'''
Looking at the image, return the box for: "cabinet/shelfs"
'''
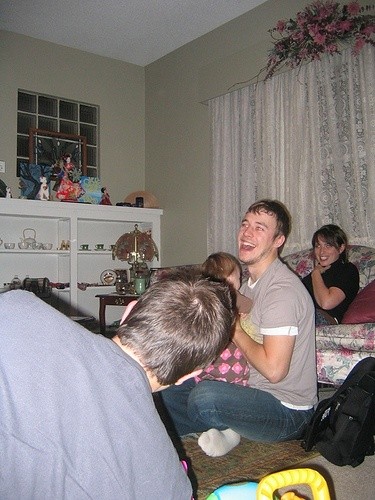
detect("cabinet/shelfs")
[0,214,161,313]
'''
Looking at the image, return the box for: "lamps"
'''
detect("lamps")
[114,221,160,287]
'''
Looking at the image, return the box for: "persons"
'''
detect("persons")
[55,153,86,203]
[100,187,111,205]
[196,251,256,387]
[0,269,233,500]
[153,199,318,456]
[297,224,361,326]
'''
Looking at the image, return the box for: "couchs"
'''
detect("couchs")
[147,244,375,386]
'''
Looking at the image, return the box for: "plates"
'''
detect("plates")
[100,270,117,285]
[108,249,114,251]
[79,249,91,250]
[94,249,106,251]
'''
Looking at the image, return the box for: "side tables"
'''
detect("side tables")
[95,293,138,335]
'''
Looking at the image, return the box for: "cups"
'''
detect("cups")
[130,285,136,294]
[117,284,125,295]
[4,283,10,291]
[136,197,143,208]
[80,245,88,250]
[135,278,145,295]
[109,245,115,249]
[95,244,104,249]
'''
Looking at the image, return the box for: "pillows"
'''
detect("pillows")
[342,279,375,325]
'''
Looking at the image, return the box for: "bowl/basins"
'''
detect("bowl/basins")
[32,242,42,249]
[4,243,15,249]
[18,242,29,249]
[42,243,52,250]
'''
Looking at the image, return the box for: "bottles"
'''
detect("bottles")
[23,275,30,288]
[66,240,71,250]
[12,275,21,285]
[61,240,66,250]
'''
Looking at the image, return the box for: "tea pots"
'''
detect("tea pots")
[19,228,37,249]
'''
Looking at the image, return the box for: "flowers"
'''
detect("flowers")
[256,0,375,80]
